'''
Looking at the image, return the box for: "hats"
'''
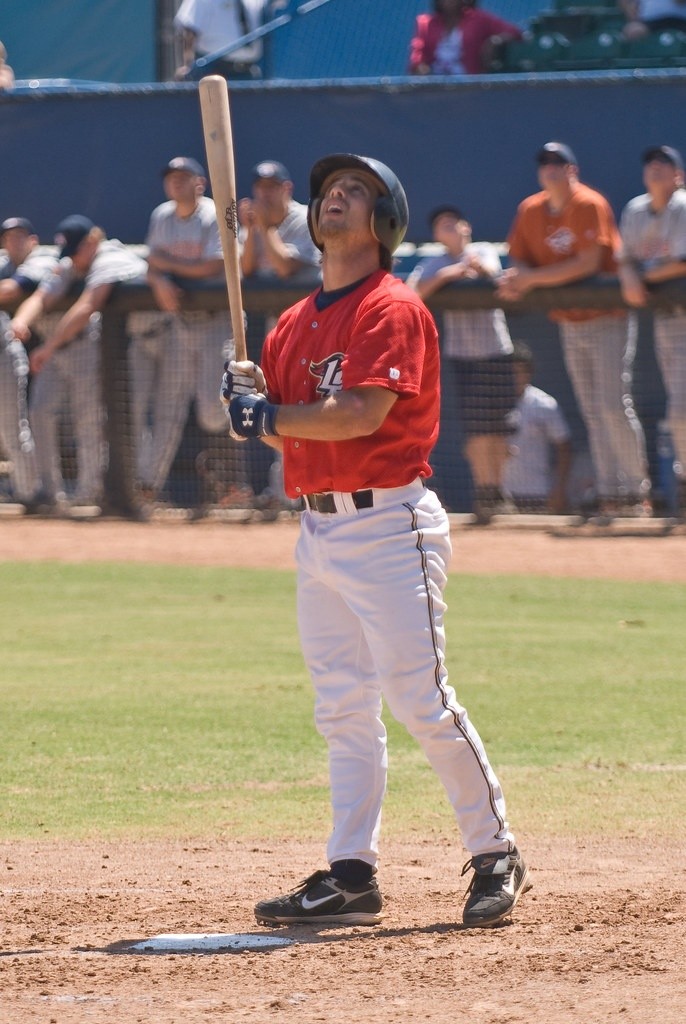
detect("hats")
[252,161,291,183]
[0,217,35,237]
[535,142,578,166]
[306,152,409,256]
[641,143,683,169]
[161,158,204,178]
[53,214,95,260]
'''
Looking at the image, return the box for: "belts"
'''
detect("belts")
[300,476,425,513]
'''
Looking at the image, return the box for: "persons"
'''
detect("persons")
[624,0,686,37]
[497,142,651,519]
[404,202,521,510]
[231,160,329,515]
[172,0,267,82]
[615,144,686,503]
[498,344,572,515]
[219,153,534,929]
[0,157,234,515]
[407,0,522,75]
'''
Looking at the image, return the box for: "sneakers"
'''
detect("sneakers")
[460,845,533,927]
[253,870,384,926]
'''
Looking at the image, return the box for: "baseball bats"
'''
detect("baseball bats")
[197,75,255,442]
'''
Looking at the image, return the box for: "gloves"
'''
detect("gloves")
[224,393,278,441]
[219,360,268,400]
